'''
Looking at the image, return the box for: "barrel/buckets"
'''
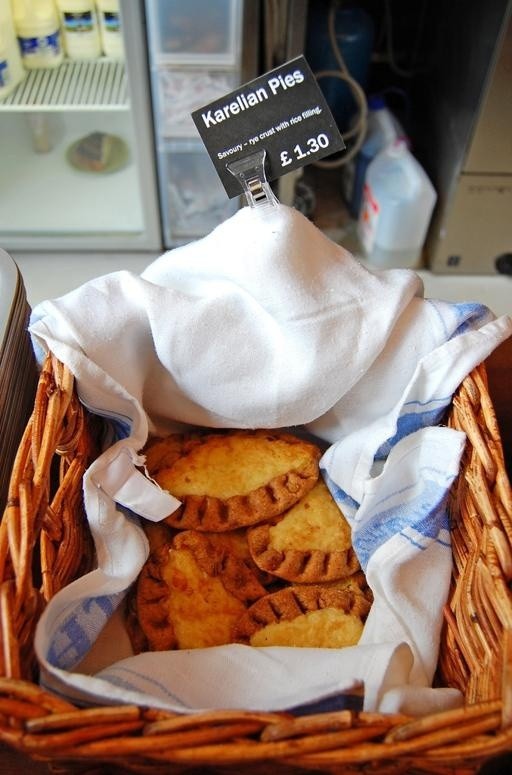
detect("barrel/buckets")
[358,141,435,276]
[311,0,376,103]
[339,103,405,218]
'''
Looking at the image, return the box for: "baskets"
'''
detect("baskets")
[1,349,511,775]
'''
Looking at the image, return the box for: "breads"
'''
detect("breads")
[124,428,374,654]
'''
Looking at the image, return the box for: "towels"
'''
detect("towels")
[25,200,512,714]
